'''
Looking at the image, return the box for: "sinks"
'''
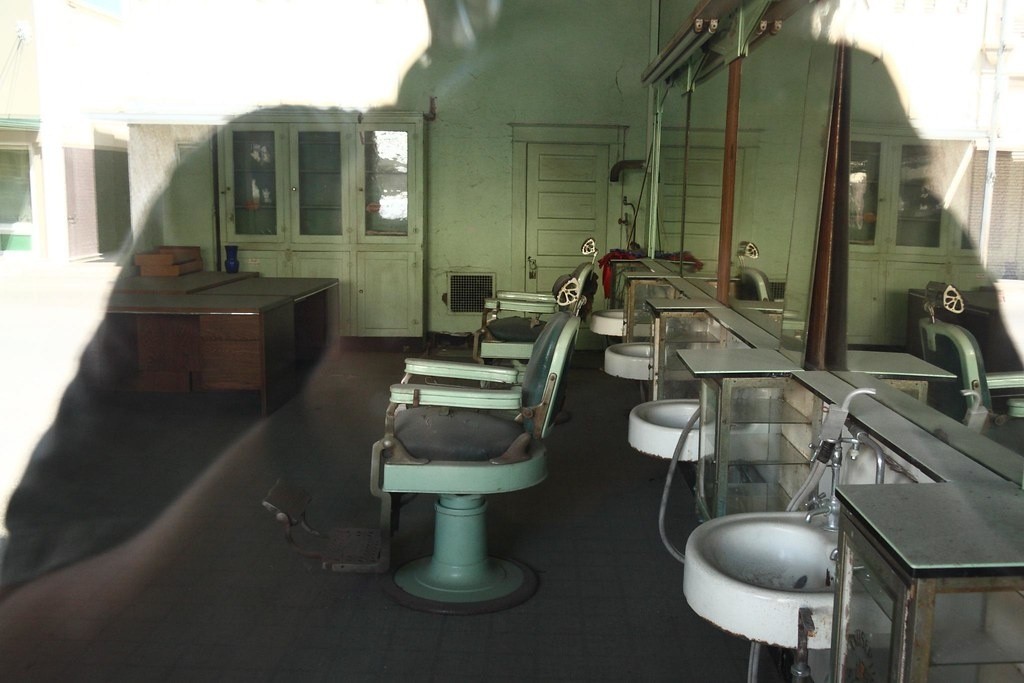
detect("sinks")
[589,309,650,337]
[604,342,693,380]
[627,398,769,462]
[681,509,950,650]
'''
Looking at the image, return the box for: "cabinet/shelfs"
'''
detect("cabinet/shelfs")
[829,481,1024,683]
[844,125,976,350]
[646,299,720,401]
[218,111,430,351]
[609,255,639,310]
[677,349,814,524]
[622,271,671,342]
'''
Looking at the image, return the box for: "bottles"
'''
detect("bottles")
[224,244,239,274]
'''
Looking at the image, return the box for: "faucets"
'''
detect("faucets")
[803,492,836,523]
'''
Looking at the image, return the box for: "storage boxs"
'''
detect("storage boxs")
[140,258,203,277]
[134,246,201,266]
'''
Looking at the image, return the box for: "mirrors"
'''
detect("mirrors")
[653,63,689,275]
[825,0,1024,488]
[681,64,730,300]
[727,0,824,339]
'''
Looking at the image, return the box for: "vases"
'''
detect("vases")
[224,245,239,272]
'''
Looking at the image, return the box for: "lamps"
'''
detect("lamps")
[640,18,719,89]
[680,19,783,97]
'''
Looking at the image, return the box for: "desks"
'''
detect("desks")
[76,292,296,419]
[76,272,261,395]
[192,277,342,396]
[902,286,1022,378]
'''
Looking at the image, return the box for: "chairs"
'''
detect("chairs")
[278,312,580,616]
[919,317,1024,434]
[472,268,598,390]
[738,267,773,302]
[392,262,593,419]
